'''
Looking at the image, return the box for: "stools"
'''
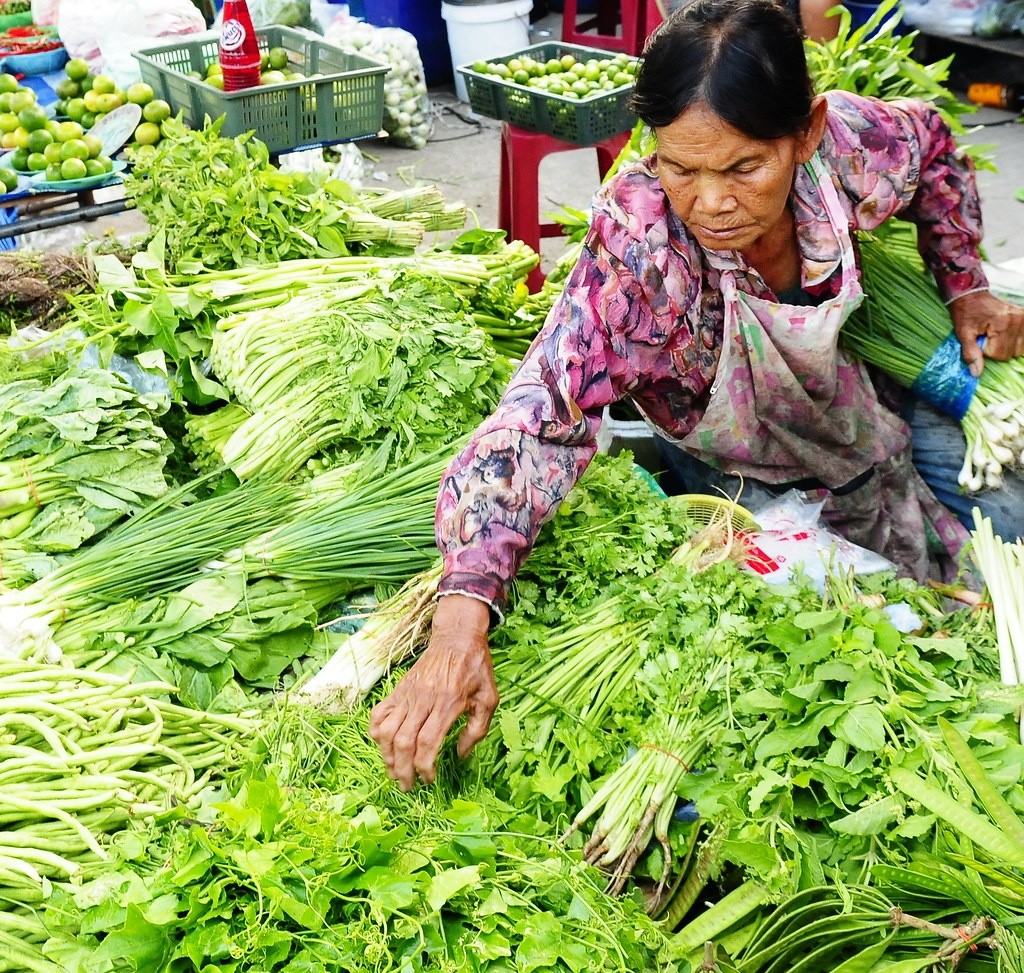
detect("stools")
[560,0,647,57]
[495,121,633,296]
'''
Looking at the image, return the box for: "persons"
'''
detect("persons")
[367,1,1024,794]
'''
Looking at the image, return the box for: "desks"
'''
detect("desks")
[0,66,152,239]
[913,30,1024,65]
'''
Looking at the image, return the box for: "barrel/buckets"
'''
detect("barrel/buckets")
[844,0,906,44]
[441,0,534,103]
[363,0,463,90]
[327,0,364,23]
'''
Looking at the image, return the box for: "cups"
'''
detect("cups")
[967,84,1012,108]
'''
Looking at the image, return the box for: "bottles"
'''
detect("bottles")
[220,0,262,120]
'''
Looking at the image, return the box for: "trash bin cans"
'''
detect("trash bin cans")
[841,0,909,47]
[441,0,535,105]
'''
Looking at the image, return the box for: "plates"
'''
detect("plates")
[86,103,143,155]
[0,152,45,175]
[116,151,137,164]
[0,175,33,201]
[31,161,126,189]
